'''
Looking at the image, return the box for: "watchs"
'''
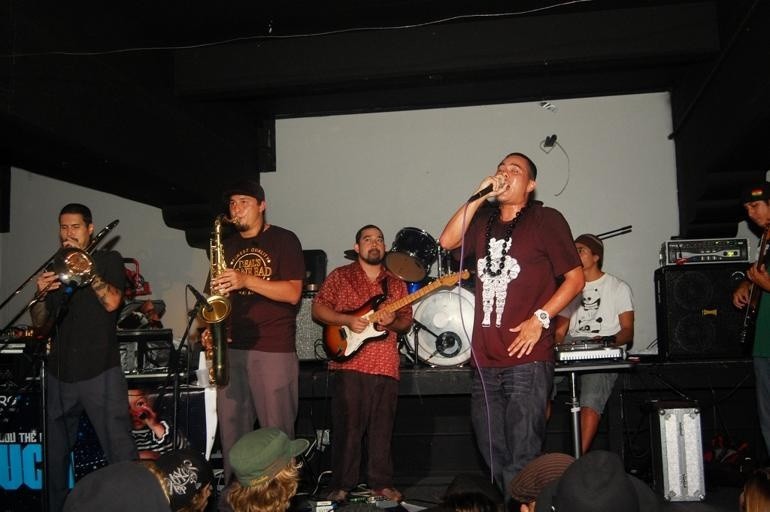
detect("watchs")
[533,308,551,330]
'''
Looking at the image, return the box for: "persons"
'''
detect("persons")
[29,202,139,512]
[217,427,311,512]
[201,181,307,487]
[310,224,414,501]
[731,181,770,453]
[439,152,586,488]
[547,233,636,454]
[509,453,576,512]
[127,385,192,462]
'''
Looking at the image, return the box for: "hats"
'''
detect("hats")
[533,450,659,512]
[228,426,310,489]
[222,180,265,201]
[574,233,605,259]
[508,452,576,503]
[743,181,770,202]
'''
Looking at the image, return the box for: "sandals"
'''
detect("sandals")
[371,486,402,502]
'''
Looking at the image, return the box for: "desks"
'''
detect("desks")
[294,372,764,478]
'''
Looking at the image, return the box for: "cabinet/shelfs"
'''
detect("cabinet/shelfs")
[116,329,182,491]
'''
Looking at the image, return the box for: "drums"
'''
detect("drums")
[384,226,437,282]
[403,285,475,367]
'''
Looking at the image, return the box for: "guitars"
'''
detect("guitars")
[738,236,770,351]
[322,269,470,363]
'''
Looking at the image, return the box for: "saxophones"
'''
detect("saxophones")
[199,213,240,388]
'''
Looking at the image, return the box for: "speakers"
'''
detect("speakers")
[653,264,756,361]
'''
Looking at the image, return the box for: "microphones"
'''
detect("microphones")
[185,284,214,315]
[63,275,82,295]
[466,184,494,206]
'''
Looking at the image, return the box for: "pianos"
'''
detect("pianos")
[552,339,634,372]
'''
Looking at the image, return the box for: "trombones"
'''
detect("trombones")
[0,218,120,352]
